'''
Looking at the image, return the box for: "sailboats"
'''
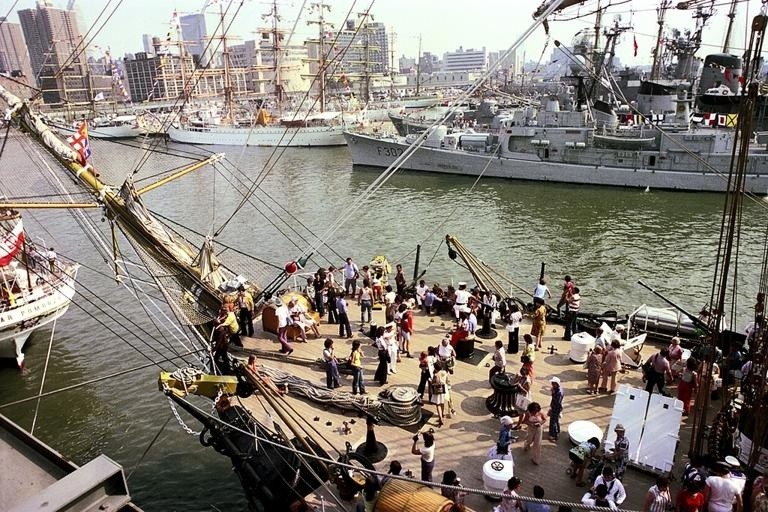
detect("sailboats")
[55,1,767,192]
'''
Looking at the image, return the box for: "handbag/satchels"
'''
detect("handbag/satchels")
[346,359,357,370]
[516,390,531,411]
[431,377,445,394]
[642,363,654,378]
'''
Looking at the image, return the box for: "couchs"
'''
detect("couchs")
[262,292,320,340]
[371,476,473,511]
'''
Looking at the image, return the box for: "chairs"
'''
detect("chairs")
[232,357,266,388]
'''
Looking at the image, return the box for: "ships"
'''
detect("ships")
[0,197,76,363]
[1,2,766,511]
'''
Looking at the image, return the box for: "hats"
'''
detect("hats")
[275,299,282,305]
[550,377,560,385]
[614,424,625,431]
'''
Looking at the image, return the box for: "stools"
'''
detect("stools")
[453,335,475,358]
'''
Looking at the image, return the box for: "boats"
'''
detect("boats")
[0,410,145,512]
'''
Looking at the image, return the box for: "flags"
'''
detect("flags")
[65,119,92,161]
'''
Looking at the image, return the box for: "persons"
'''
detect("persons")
[25,246,58,296]
[217,257,768,512]
